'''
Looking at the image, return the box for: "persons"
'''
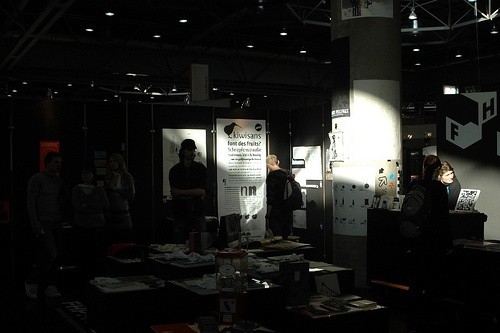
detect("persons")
[265,155,294,240]
[407,155,461,316]
[26,151,135,298]
[169,139,209,248]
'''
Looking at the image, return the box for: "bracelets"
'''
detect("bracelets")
[265,214,269,219]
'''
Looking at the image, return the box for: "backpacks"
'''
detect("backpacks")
[401,177,438,239]
[269,169,304,209]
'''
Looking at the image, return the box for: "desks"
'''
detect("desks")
[365,207,488,291]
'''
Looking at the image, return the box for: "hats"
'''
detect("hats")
[181,139,197,149]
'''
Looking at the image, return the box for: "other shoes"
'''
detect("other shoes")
[24,280,38,298]
[44,286,61,297]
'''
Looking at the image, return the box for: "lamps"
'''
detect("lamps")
[408,6,418,19]
